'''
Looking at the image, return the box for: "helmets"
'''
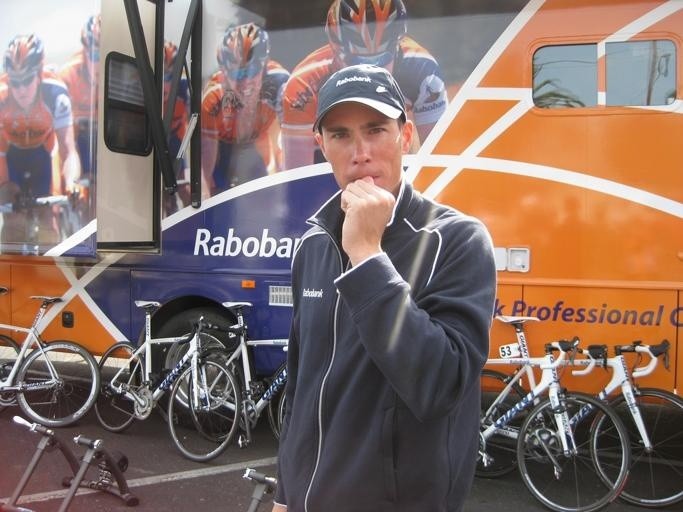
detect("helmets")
[325,0,408,55]
[164,41,177,66]
[81,15,100,46]
[216,22,270,68]
[3,32,44,76]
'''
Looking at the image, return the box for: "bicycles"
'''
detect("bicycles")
[4,193,86,259]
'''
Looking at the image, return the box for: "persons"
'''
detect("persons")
[162,42,191,216]
[201,23,289,196]
[272,65,496,512]
[282,0,448,168]
[0,15,100,243]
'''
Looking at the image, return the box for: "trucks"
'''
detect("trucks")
[1,0,683,437]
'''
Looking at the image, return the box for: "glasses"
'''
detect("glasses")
[227,69,261,80]
[10,77,35,88]
[164,72,173,81]
[89,51,100,62]
[344,52,392,66]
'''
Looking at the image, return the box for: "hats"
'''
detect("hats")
[313,64,407,133]
